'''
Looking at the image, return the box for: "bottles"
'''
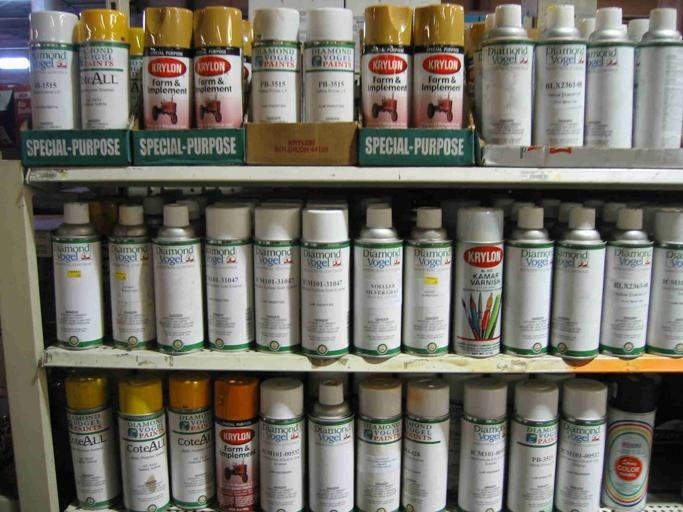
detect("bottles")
[62,369,660,512]
[52,193,682,359]
[27,2,682,151]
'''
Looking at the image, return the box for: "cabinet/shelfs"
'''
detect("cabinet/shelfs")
[0,145,683,508]
